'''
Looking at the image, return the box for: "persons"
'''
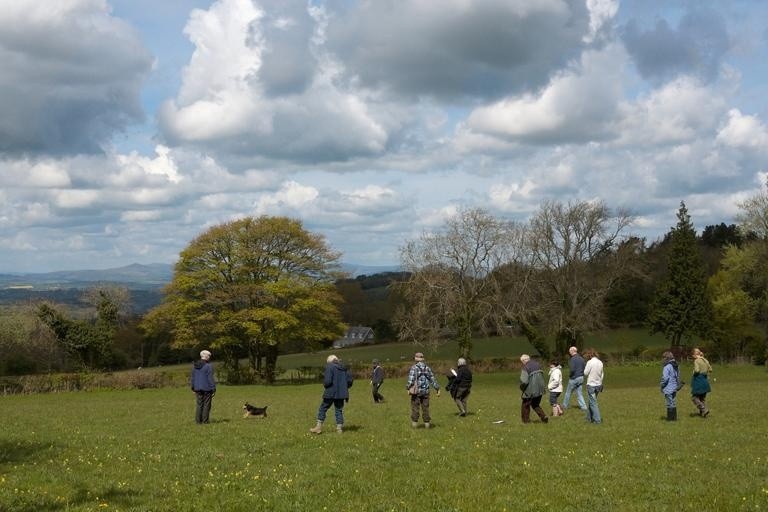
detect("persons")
[370,358,384,403]
[406,352,441,429]
[690,348,712,417]
[519,354,548,424]
[584,347,604,424]
[446,357,472,416]
[191,350,215,424]
[310,355,353,434]
[563,346,588,410]
[547,359,564,417]
[660,351,679,421]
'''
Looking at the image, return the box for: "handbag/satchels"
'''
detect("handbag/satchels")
[407,383,419,395]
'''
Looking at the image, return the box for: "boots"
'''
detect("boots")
[425,423,431,429]
[309,420,323,434]
[412,421,418,429]
[335,424,343,434]
[666,408,678,421]
[551,404,563,417]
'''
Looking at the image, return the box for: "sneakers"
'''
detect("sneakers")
[701,408,710,417]
[542,416,549,424]
[458,409,468,417]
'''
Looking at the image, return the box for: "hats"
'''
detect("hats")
[326,355,338,364]
[415,352,425,361]
[457,358,467,367]
[519,353,530,361]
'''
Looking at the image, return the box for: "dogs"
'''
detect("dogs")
[242,401,268,420]
